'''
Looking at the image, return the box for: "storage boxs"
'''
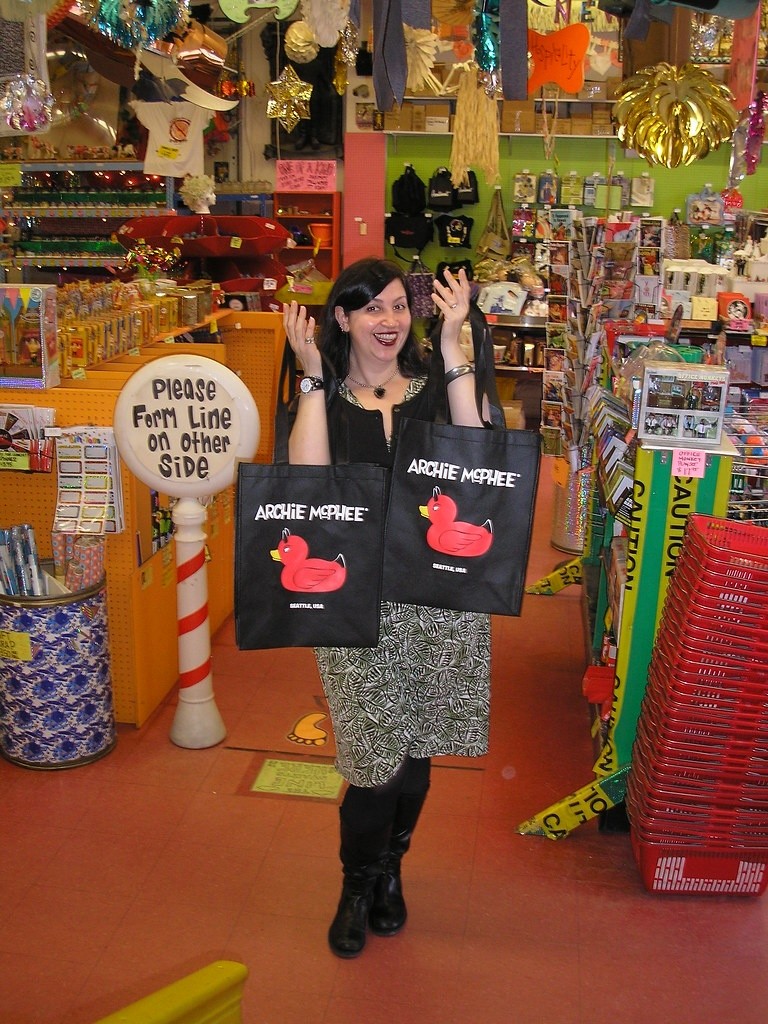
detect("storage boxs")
[577,80,608,100]
[666,290,692,319]
[412,105,425,131]
[691,296,719,322]
[425,116,449,132]
[405,61,483,97]
[569,103,592,119]
[592,104,613,136]
[501,101,535,133]
[542,82,576,99]
[552,119,571,135]
[718,292,752,319]
[729,259,768,303]
[0,283,61,389]
[637,360,730,444]
[426,101,451,117]
[571,118,592,135]
[496,377,526,429]
[535,113,553,134]
[383,103,412,132]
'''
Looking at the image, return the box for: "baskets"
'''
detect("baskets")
[625,513,768,900]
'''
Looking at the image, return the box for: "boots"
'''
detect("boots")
[368,793,426,937]
[327,807,395,959]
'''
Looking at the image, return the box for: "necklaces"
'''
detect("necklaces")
[347,365,400,399]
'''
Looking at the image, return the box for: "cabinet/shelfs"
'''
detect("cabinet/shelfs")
[517,346,768,842]
[174,193,274,218]
[0,159,174,268]
[382,96,619,155]
[273,190,342,282]
[112,215,293,312]
[0,308,287,730]
[483,312,548,372]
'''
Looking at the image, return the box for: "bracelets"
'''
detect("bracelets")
[445,363,476,386]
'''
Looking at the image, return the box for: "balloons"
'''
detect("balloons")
[0,74,55,132]
[265,65,314,133]
[612,62,741,170]
[727,90,768,190]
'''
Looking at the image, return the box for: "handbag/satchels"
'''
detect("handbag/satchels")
[234,337,387,656]
[392,165,426,216]
[434,215,474,248]
[384,212,433,263]
[402,258,436,319]
[474,190,512,261]
[427,166,478,212]
[380,301,541,616]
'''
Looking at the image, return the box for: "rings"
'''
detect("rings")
[450,303,457,309]
[305,337,314,344]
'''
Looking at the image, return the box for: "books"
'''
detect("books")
[562,213,666,528]
[540,209,577,457]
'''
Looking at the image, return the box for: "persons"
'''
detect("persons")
[282,254,492,958]
[684,415,718,438]
[645,412,678,435]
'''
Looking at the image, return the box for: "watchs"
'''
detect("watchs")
[298,375,327,395]
